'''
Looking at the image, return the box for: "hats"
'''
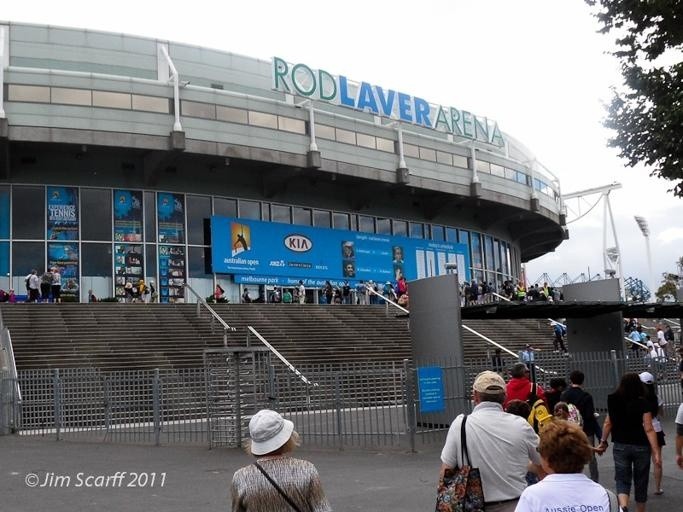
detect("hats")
[473,371,508,395]
[639,372,654,385]
[550,321,557,326]
[249,410,294,456]
[510,361,531,372]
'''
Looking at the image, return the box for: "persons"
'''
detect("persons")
[439,370,547,512]
[393,246,404,264]
[233,226,247,250]
[230,408,333,512]
[344,261,355,277]
[343,241,354,258]
[504,317,683,512]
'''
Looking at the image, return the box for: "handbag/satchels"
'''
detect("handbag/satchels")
[435,464,487,512]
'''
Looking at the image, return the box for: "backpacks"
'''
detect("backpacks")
[553,400,583,429]
[560,324,566,335]
[525,392,552,435]
